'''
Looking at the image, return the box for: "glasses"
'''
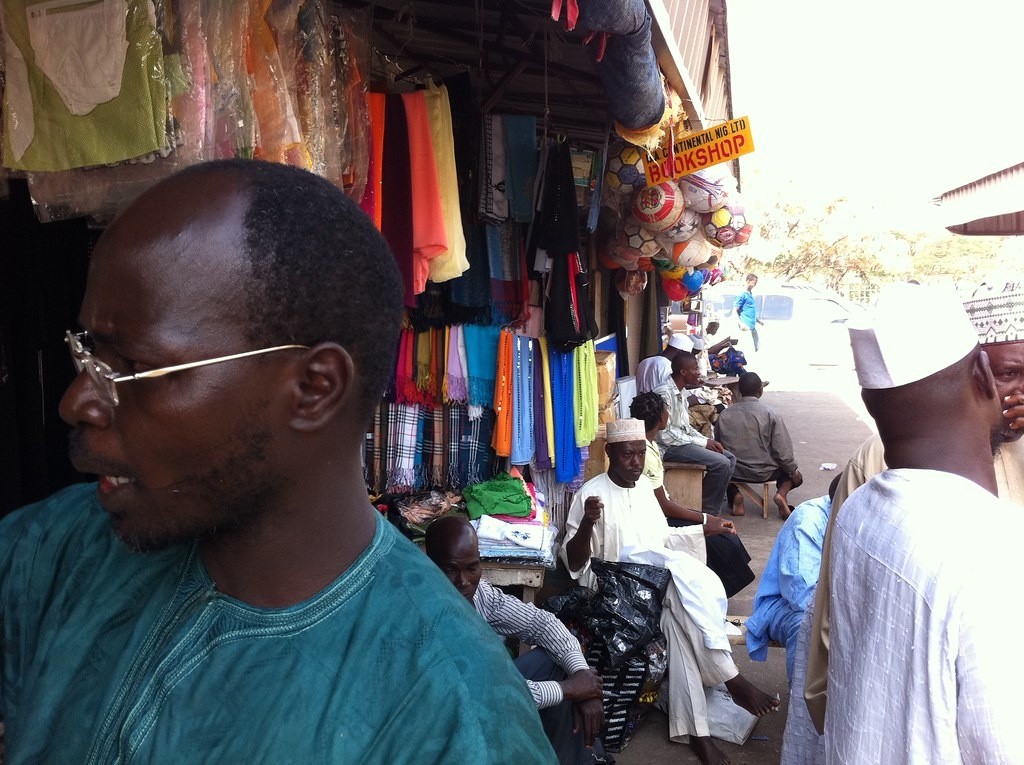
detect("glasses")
[64,328,312,407]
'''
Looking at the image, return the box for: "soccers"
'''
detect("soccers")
[603,140,757,268]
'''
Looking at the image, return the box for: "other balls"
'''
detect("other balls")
[681,269,704,290]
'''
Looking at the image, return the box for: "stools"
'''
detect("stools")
[729,481,777,519]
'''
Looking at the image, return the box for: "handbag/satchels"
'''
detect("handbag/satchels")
[589,556,671,671]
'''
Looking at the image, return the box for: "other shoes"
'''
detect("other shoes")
[762,381,769,387]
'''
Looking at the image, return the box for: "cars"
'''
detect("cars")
[727,289,860,368]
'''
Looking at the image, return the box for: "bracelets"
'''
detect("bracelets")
[701,512,709,525]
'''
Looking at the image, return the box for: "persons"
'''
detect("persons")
[422,514,605,763]
[825,285,1023,764]
[557,332,844,765]
[808,272,1023,729]
[732,274,764,353]
[0,158,561,765]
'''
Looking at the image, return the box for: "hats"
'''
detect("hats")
[688,335,703,350]
[848,282,978,389]
[606,418,647,443]
[962,283,1024,345]
[668,333,694,353]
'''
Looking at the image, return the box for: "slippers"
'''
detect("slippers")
[782,505,795,521]
